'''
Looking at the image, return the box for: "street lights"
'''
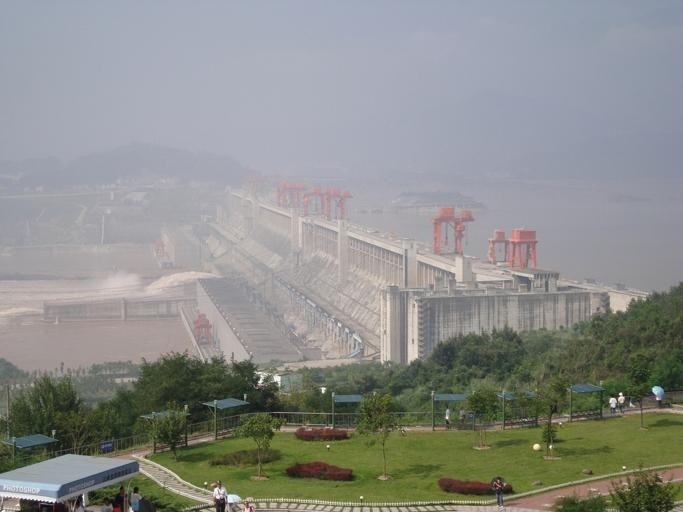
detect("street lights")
[471,389,477,432]
[598,380,605,419]
[150,410,157,454]
[242,392,249,402]
[331,390,336,429]
[11,436,17,465]
[430,390,436,431]
[51,429,57,438]
[183,404,188,447]
[213,399,217,440]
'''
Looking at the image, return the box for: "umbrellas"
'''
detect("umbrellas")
[652,385,664,397]
[225,494,241,503]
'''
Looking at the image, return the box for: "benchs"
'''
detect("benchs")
[153,427,237,453]
[433,408,602,431]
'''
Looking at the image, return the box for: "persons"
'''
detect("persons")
[116,486,131,511]
[608,394,618,416]
[225,502,235,511]
[459,407,465,425]
[445,405,452,429]
[129,487,142,512]
[618,391,626,415]
[213,480,228,511]
[493,478,505,508]
[242,501,255,512]
[656,396,662,408]
[112,502,122,512]
[73,495,85,512]
[101,497,114,512]
